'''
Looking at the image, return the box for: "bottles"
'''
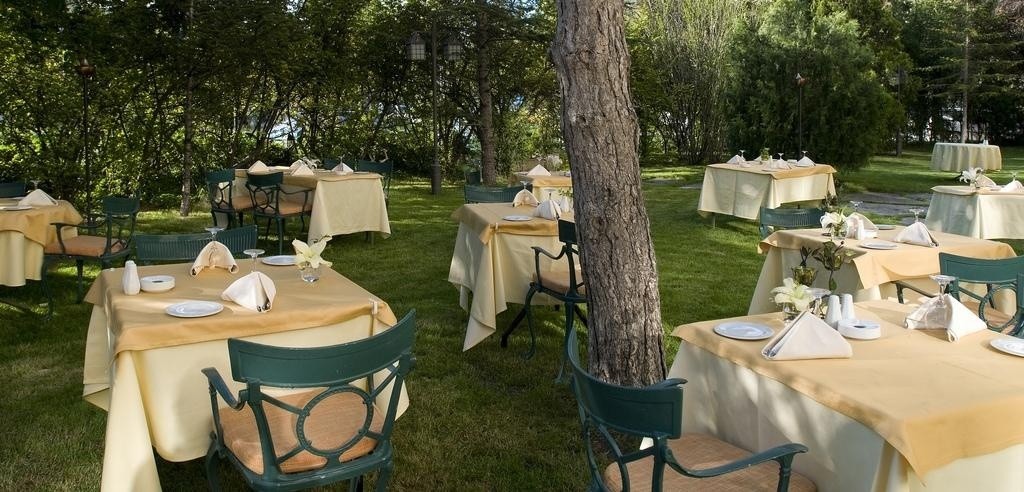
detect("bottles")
[121,259,141,296]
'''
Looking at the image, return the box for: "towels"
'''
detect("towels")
[247,160,268,173]
[289,160,308,169]
[190,240,238,275]
[331,163,354,175]
[19,188,58,207]
[290,164,314,176]
[221,272,277,312]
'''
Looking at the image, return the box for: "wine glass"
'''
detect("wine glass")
[928,274,957,337]
[29,179,41,191]
[909,208,926,225]
[1009,170,1019,183]
[337,155,346,164]
[844,199,866,238]
[982,132,990,145]
[204,226,226,273]
[739,149,746,160]
[543,188,556,201]
[770,151,808,160]
[242,249,266,276]
[536,157,543,166]
[807,286,831,320]
[301,240,322,288]
[519,180,530,190]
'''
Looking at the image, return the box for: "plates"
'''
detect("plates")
[761,167,785,173]
[854,240,902,251]
[0,205,33,211]
[165,299,223,319]
[317,168,331,174]
[353,170,371,175]
[712,321,776,341]
[502,214,534,222]
[836,317,882,341]
[871,222,897,231]
[260,254,307,266]
[138,274,176,294]
[988,335,1024,361]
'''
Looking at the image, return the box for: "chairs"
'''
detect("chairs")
[198,306,417,491]
[41,190,141,319]
[246,171,314,254]
[0,181,27,197]
[203,166,253,226]
[136,225,257,266]
[324,158,355,170]
[357,158,393,211]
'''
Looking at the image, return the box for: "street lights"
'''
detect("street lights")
[407,17,461,195]
[888,63,909,159]
[74,57,97,236]
[794,73,807,162]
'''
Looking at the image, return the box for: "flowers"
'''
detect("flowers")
[291,236,332,269]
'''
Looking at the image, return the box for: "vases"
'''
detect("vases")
[299,262,320,283]
